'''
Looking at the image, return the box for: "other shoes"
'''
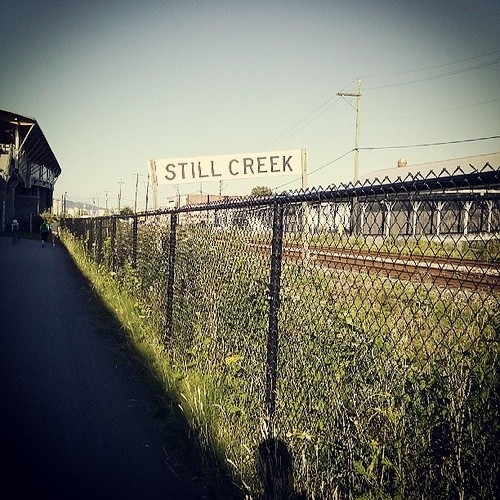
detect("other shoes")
[42,244,45,247]
[51,244,56,247]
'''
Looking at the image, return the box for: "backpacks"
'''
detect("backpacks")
[13,224,18,231]
[41,227,48,233]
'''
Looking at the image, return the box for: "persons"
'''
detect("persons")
[40,220,50,247]
[12,219,19,242]
[50,217,60,247]
